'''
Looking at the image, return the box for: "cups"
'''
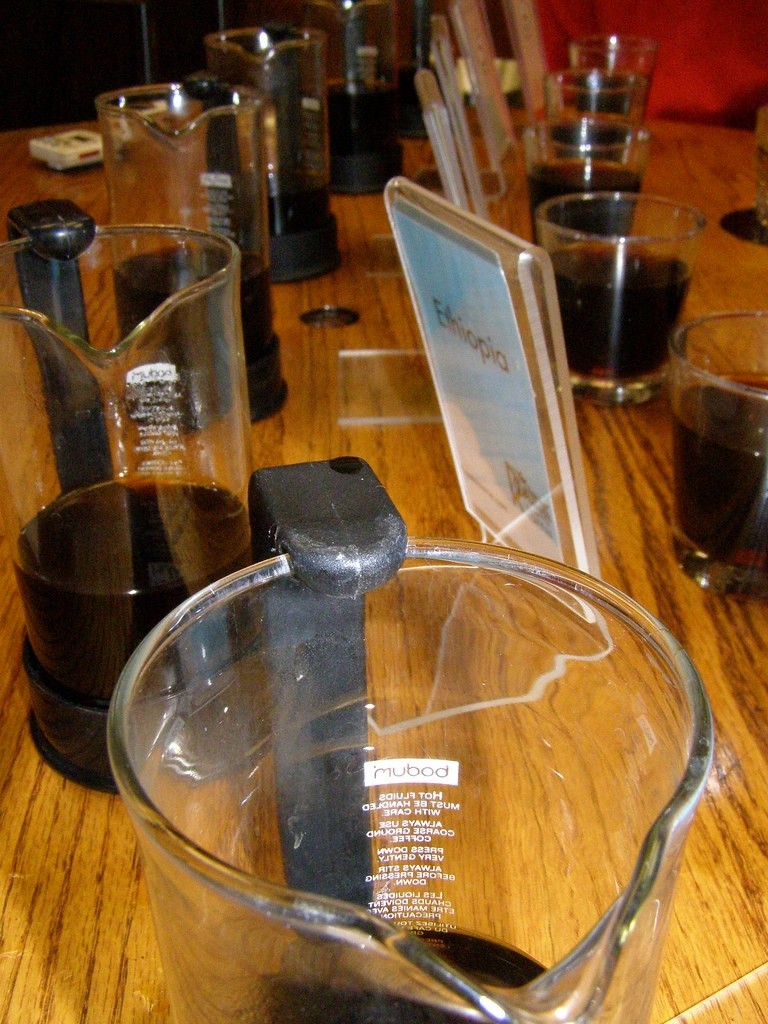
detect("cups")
[666,311,768,594]
[524,34,704,404]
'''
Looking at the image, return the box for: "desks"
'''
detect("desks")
[0,115,766,1024]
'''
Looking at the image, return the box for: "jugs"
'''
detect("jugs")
[110,458,712,1024]
[0,0,427,794]
